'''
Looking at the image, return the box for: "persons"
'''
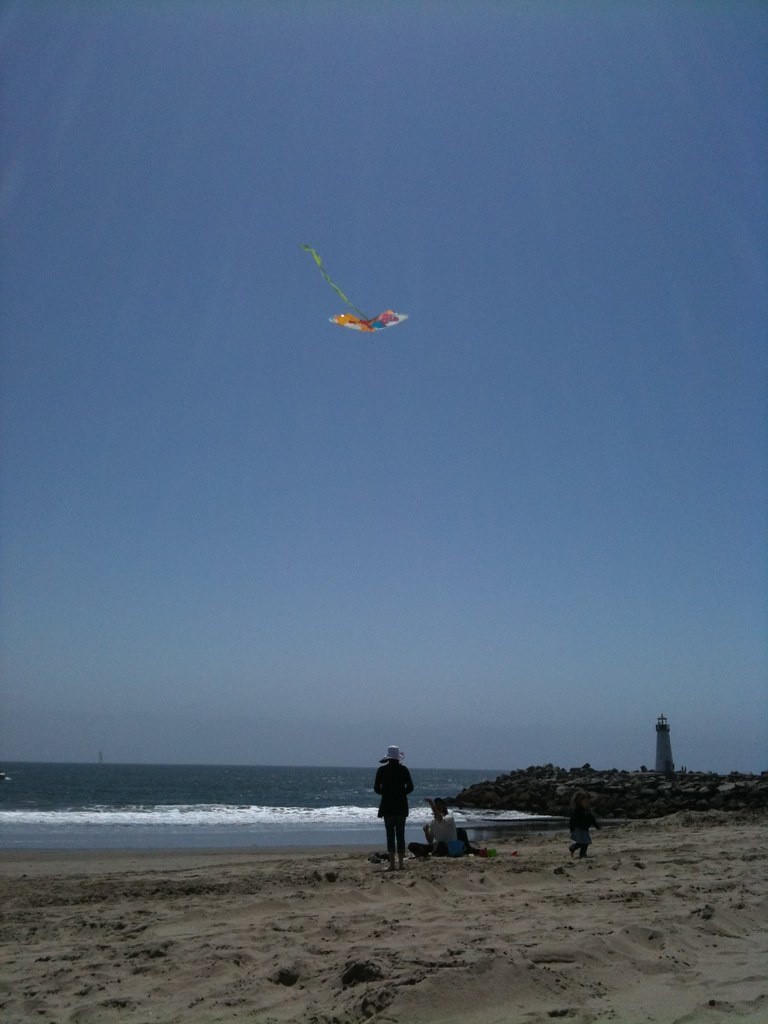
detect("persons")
[567,792,602,858]
[374,743,415,872]
[409,798,458,857]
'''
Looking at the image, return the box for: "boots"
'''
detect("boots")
[398,852,406,870]
[385,852,396,871]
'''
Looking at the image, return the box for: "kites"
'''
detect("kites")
[303,244,410,334]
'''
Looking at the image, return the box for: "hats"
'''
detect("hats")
[574,793,591,803]
[379,745,405,763]
[434,797,449,807]
